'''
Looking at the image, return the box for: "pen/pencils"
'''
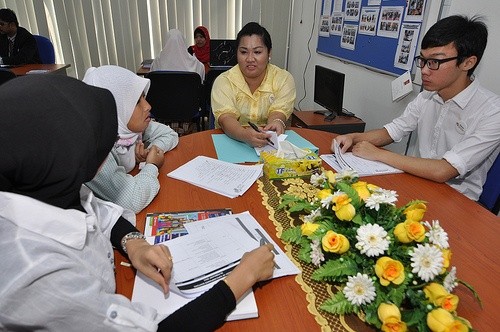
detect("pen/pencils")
[260,237,265,289]
[248,121,274,145]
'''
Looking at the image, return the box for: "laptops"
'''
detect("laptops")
[208,39,239,68]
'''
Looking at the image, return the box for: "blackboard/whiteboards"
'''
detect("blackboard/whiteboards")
[315,0,445,81]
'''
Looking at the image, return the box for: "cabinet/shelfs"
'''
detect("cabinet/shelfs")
[291,110,366,134]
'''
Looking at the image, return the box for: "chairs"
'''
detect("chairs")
[145,69,231,133]
[33,35,55,65]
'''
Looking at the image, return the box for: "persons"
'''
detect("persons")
[0,73,275,332]
[150,29,205,84]
[0,8,42,67]
[81,65,179,213]
[320,0,424,66]
[330,15,500,200]
[211,22,297,147]
[187,26,212,74]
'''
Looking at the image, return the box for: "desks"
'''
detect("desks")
[0,63,71,77]
[135,57,210,77]
[111,128,500,332]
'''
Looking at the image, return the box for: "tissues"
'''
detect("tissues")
[259,140,322,180]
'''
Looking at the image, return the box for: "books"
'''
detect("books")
[130,133,404,321]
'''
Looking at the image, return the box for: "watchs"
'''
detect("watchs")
[122,232,146,253]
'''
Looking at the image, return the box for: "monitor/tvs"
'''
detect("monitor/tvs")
[314,65,345,116]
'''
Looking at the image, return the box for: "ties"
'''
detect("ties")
[8,39,17,56]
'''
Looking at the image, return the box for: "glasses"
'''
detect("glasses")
[413,55,459,71]
[0,22,6,26]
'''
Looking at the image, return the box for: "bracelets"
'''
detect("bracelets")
[272,119,286,128]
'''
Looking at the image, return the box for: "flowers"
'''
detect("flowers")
[278,170,485,332]
